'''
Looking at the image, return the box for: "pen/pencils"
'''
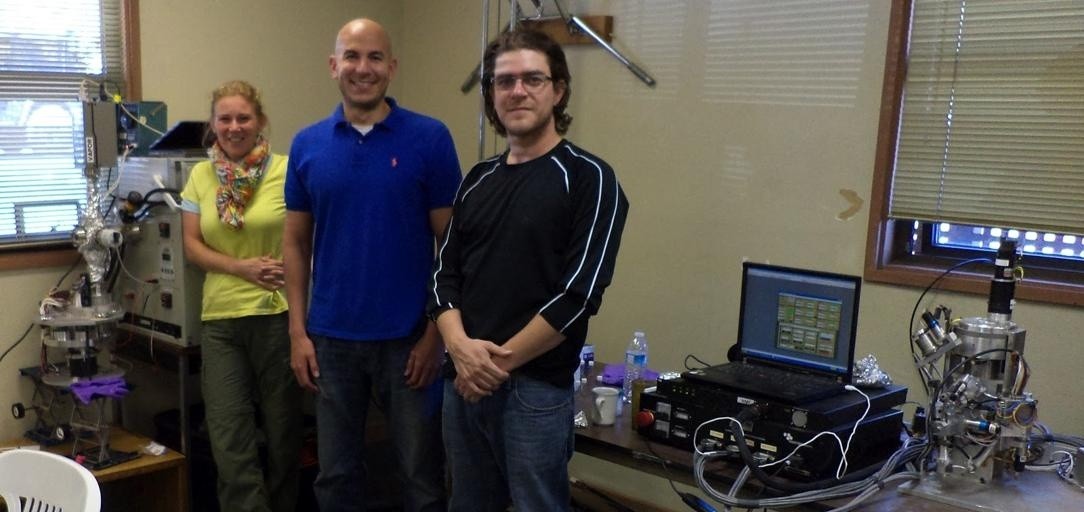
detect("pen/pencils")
[616,390,624,417]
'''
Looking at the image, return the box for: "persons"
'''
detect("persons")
[422,31,629,508]
[280,20,465,509]
[174,78,318,512]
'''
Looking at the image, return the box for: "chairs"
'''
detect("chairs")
[0,449,104,512]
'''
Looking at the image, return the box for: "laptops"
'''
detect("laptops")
[148,120,209,157]
[680,260,862,404]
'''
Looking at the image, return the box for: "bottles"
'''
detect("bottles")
[623,331,649,402]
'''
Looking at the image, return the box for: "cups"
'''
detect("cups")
[590,387,619,426]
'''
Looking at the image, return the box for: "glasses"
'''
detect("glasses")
[491,75,552,89]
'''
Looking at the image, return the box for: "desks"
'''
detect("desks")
[575,360,1084,512]
[0,422,189,512]
